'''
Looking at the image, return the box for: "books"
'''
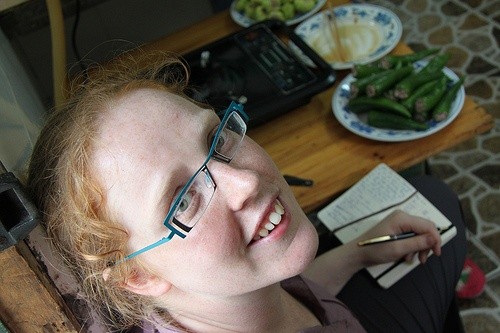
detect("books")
[316,163,458,290]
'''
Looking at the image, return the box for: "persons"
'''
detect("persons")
[28,47,469,333]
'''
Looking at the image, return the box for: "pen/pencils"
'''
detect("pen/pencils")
[356,227,441,247]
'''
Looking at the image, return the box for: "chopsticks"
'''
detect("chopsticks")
[322,2,346,64]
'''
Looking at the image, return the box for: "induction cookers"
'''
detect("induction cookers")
[127,19,334,127]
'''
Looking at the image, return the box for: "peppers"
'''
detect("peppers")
[348,49,466,132]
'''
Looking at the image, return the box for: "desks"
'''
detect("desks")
[68,0,492,217]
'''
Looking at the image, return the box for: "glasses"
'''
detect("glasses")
[107,101,248,267]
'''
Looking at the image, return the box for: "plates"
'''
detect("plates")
[295,4,403,70]
[332,57,465,141]
[230,0,325,29]
